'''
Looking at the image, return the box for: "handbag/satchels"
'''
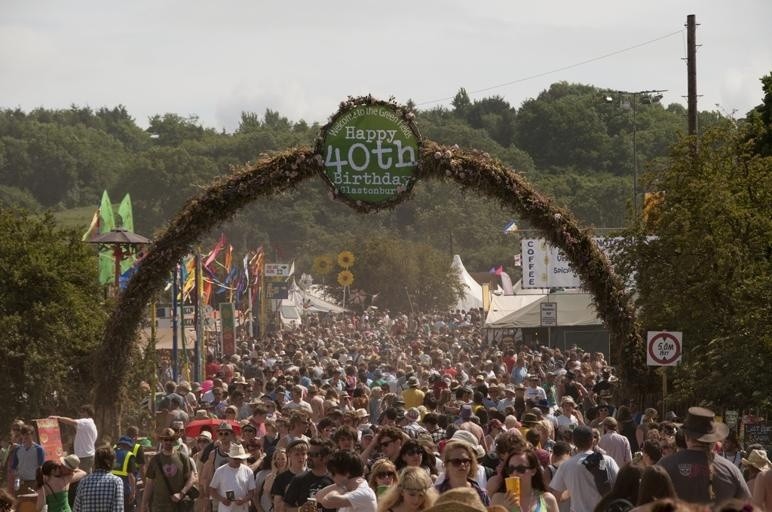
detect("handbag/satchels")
[177,495,194,510]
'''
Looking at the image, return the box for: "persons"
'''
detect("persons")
[1,357,256,511]
[212,306,619,512]
[593,403,771,512]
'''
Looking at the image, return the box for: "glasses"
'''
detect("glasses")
[377,471,394,479]
[448,458,468,466]
[508,464,530,473]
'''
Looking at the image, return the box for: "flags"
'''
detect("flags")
[481,267,513,305]
[81,190,266,304]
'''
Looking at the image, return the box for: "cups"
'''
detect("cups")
[503,477,520,507]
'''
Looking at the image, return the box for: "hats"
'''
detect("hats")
[422,487,509,512]
[118,436,133,447]
[741,449,772,471]
[60,454,80,472]
[160,346,617,459]
[673,407,729,443]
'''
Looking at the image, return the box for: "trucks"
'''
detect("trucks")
[271,298,302,332]
